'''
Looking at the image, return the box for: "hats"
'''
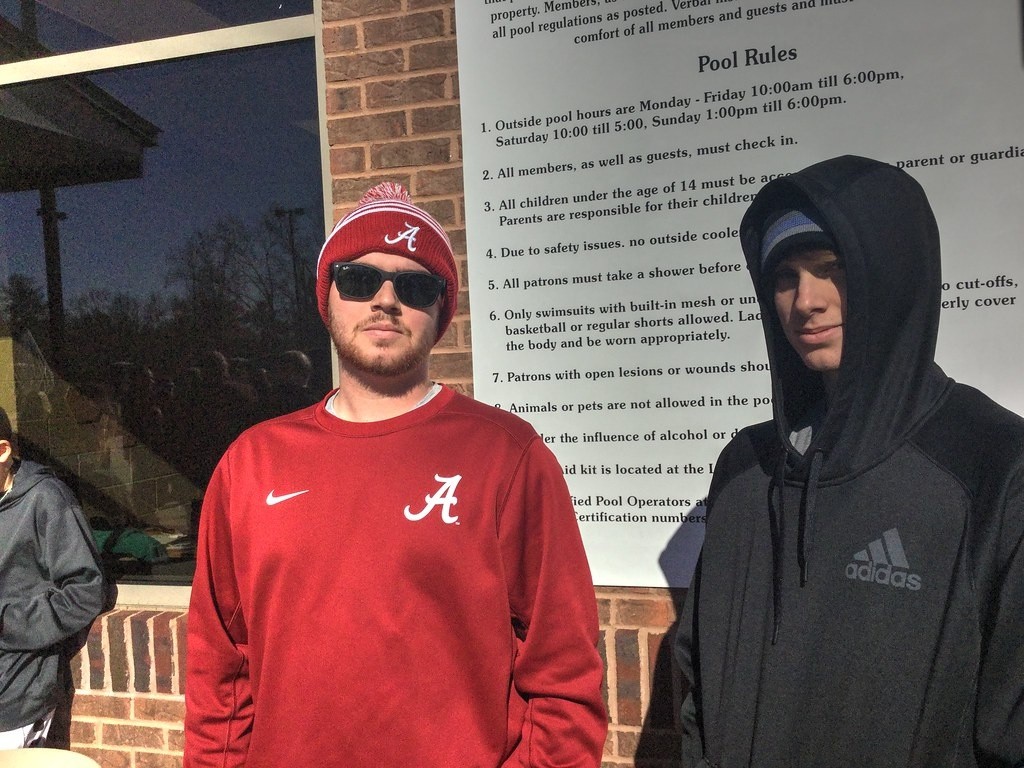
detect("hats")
[315,182,458,348]
[759,194,836,296]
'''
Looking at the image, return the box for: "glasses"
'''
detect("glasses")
[327,260,446,308]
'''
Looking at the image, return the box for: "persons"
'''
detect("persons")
[677,154,1024,767]
[113,350,321,470]
[182,182,607,768]
[0,406,105,750]
